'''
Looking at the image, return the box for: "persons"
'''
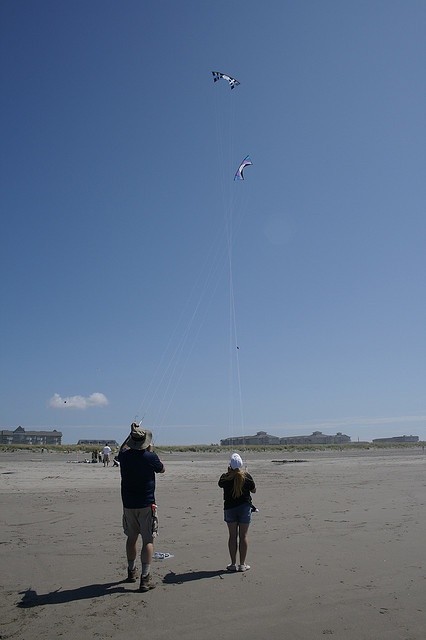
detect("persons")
[101,443,112,467]
[93,449,98,459]
[91,450,95,459]
[217,451,257,573]
[232,152,253,182]
[211,70,242,92]
[98,451,102,463]
[117,419,166,593]
[112,455,119,467]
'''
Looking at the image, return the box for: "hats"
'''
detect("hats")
[231,453,243,469]
[126,428,152,451]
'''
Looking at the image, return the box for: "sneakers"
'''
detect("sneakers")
[227,564,236,571]
[128,566,140,582]
[139,572,156,592]
[238,564,250,572]
